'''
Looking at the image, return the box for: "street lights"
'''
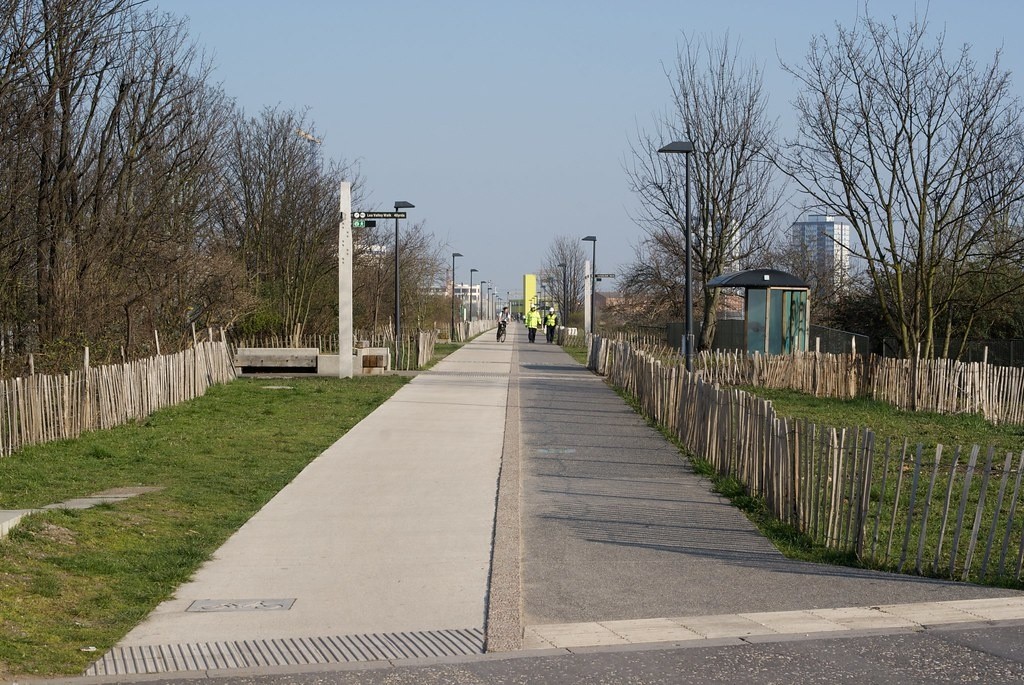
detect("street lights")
[480,281,487,320]
[537,291,542,313]
[495,295,498,319]
[451,253,464,341]
[532,298,535,305]
[488,288,493,320]
[470,268,478,321]
[534,295,538,305]
[500,299,503,311]
[547,276,554,307]
[658,140,694,375]
[394,200,416,369]
[558,263,567,327]
[540,286,546,328]
[492,293,496,320]
[582,236,597,333]
[498,298,500,310]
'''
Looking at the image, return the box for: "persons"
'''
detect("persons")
[496,307,511,342]
[544,307,558,344]
[525,302,542,343]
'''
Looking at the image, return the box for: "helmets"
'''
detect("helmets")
[530,304,535,308]
[549,307,554,312]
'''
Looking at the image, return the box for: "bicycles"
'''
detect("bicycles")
[497,323,508,343]
